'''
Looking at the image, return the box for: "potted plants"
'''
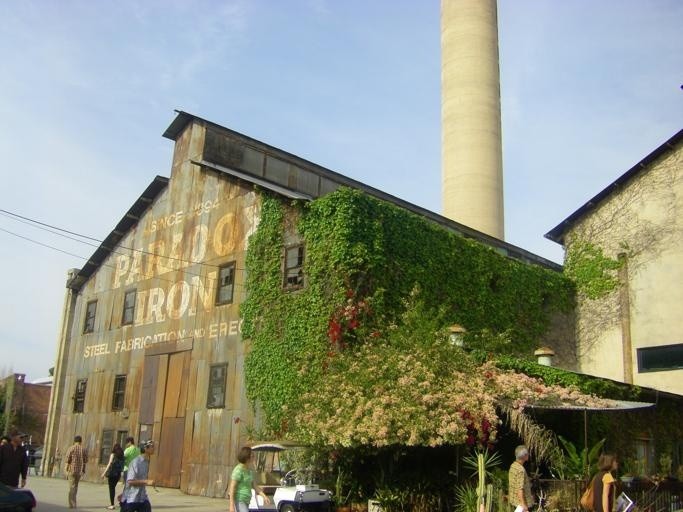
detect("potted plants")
[330,465,402,512]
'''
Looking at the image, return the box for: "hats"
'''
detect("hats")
[9,431,28,439]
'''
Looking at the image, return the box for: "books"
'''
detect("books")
[615,492,632,512]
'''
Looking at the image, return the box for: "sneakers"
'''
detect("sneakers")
[106,505,115,509]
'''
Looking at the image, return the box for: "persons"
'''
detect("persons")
[508,444,534,511]
[591,453,619,511]
[0,431,29,489]
[0,436,10,446]
[100,443,123,510]
[64,436,87,508]
[123,437,140,490]
[227,447,270,511]
[119,439,154,511]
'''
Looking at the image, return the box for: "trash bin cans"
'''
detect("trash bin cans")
[368,499,387,512]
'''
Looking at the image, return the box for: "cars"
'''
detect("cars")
[28,445,44,468]
